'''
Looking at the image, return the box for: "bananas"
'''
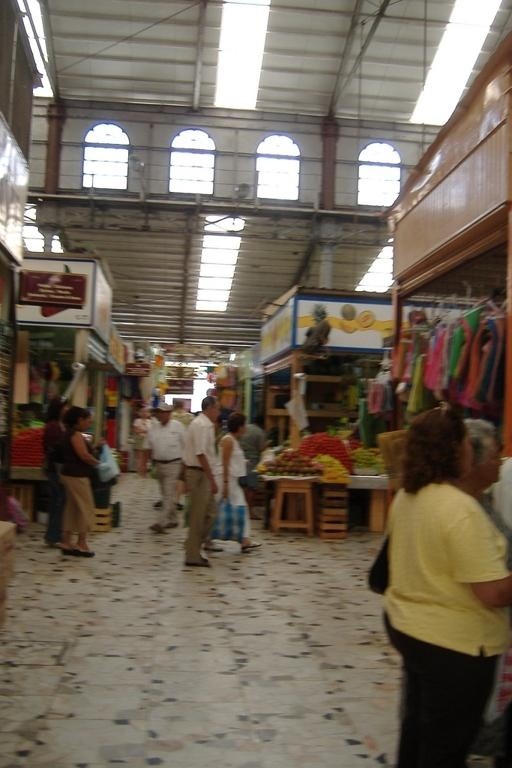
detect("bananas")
[256,464,268,473]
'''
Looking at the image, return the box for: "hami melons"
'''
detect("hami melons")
[342,304,356,320]
[358,310,374,328]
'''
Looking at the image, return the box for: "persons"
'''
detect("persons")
[41,392,72,547]
[134,397,227,512]
[204,412,262,553]
[141,399,187,533]
[55,403,107,557]
[380,404,511,768]
[456,416,511,568]
[239,415,268,522]
[180,395,219,567]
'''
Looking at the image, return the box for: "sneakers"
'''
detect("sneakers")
[149,499,183,533]
[185,542,262,567]
[43,537,95,557]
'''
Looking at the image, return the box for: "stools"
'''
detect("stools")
[267,478,315,538]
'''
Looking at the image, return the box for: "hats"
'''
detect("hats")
[153,402,175,412]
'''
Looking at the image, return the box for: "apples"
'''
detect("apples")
[350,447,377,468]
[264,449,321,476]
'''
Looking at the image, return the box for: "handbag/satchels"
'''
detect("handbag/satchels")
[96,444,120,482]
[368,535,389,593]
